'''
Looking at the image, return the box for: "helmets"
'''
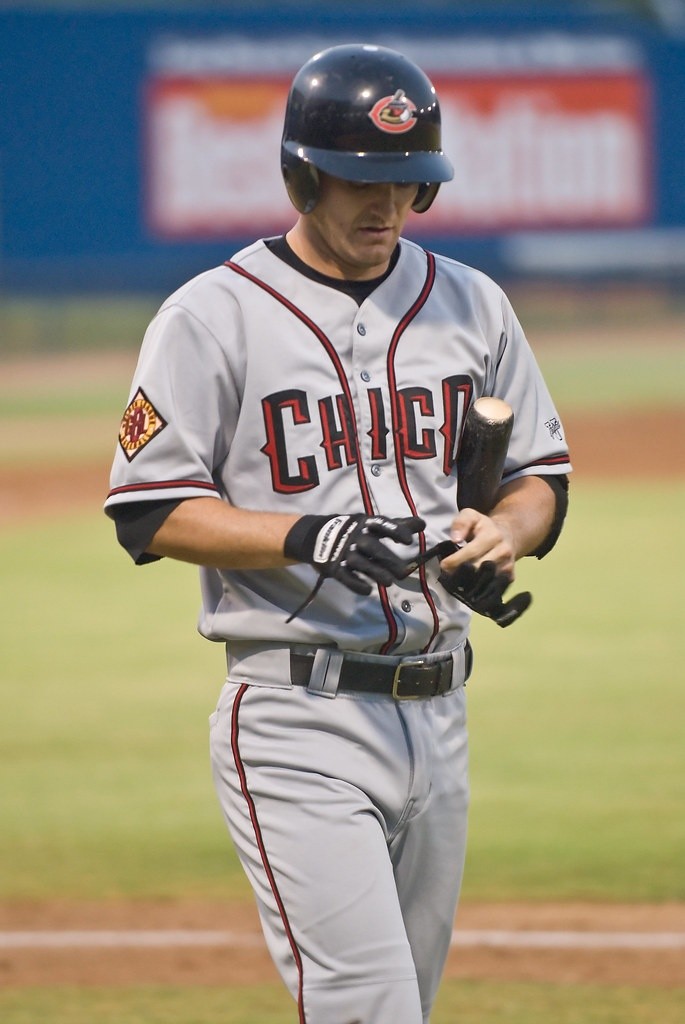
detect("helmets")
[280,43,453,214]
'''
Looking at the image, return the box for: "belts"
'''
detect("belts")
[290,637,473,701]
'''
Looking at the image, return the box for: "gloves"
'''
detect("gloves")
[397,540,531,629]
[284,514,426,596]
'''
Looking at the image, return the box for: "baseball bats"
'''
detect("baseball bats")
[458,396,514,517]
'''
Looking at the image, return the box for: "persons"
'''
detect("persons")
[103,46,577,1024]
